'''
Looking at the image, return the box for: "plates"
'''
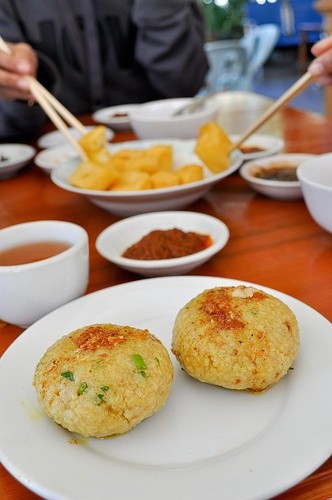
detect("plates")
[240,154,319,199]
[37,125,114,147]
[92,104,141,130]
[183,135,285,170]
[0,143,36,179]
[95,210,230,278]
[0,275,331,500]
[33,142,112,174]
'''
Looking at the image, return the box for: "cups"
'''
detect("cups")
[1,220,89,329]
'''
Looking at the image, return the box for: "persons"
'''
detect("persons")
[0,0,212,143]
[310,0,331,90]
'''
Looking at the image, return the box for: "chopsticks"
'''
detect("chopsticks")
[0,36,90,165]
[226,72,317,155]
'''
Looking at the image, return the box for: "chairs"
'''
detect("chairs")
[201,24,280,93]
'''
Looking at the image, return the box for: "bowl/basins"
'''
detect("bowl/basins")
[296,152,332,234]
[49,138,245,217]
[127,97,220,141]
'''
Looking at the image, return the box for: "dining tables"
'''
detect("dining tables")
[0,88,331,500]
